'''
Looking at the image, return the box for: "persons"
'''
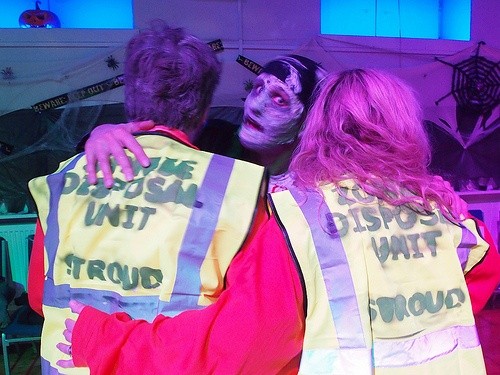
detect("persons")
[82,53,330,187]
[51,65,498,375]
[24,11,270,375]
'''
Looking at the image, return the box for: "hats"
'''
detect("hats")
[260,55,330,111]
